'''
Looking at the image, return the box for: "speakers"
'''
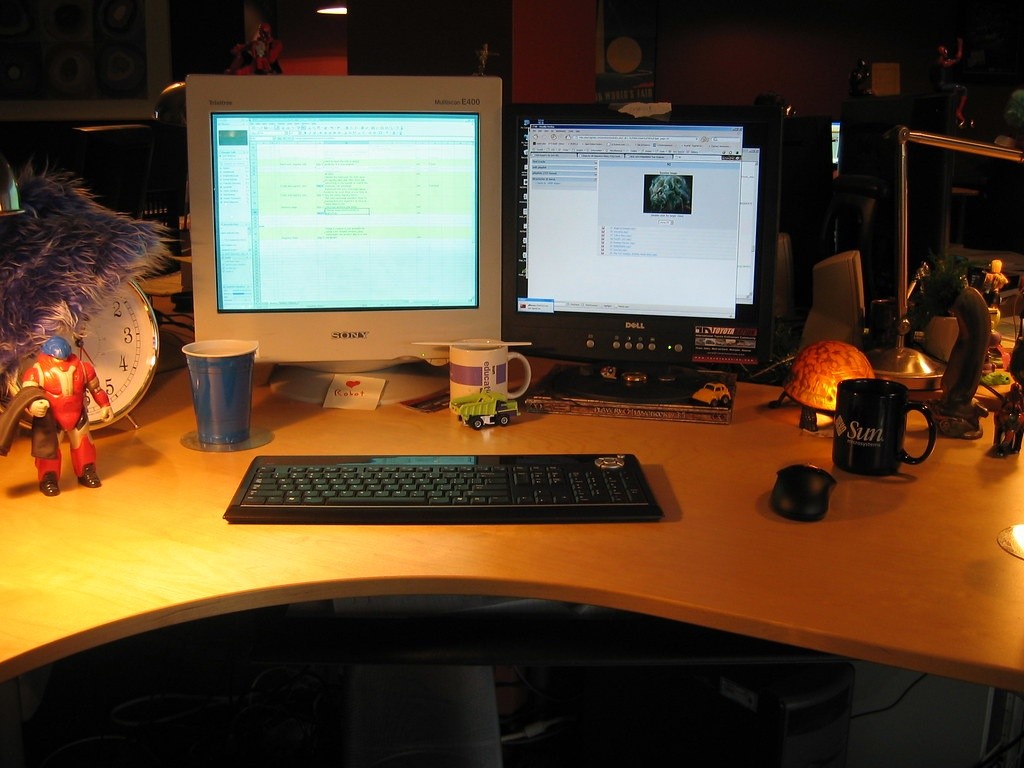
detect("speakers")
[797,251,866,356]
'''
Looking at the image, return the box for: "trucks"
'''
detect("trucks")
[449,389,521,430]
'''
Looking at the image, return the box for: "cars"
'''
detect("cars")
[690,381,732,407]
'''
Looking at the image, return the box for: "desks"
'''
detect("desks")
[0,363,1024,768]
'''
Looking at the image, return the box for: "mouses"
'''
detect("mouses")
[770,464,837,522]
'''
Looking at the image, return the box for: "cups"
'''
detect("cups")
[832,378,937,477]
[182,338,259,445]
[449,339,532,415]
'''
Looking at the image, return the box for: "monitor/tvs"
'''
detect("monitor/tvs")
[185,73,503,408]
[500,102,787,406]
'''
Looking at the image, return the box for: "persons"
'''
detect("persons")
[225,24,284,74]
[20,336,114,495]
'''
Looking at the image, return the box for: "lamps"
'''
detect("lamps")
[785,340,876,431]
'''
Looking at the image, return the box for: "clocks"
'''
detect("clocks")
[0,273,161,432]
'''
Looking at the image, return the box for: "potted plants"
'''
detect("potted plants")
[965,259,1002,331]
[922,248,975,360]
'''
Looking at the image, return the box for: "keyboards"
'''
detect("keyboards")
[223,454,664,525]
